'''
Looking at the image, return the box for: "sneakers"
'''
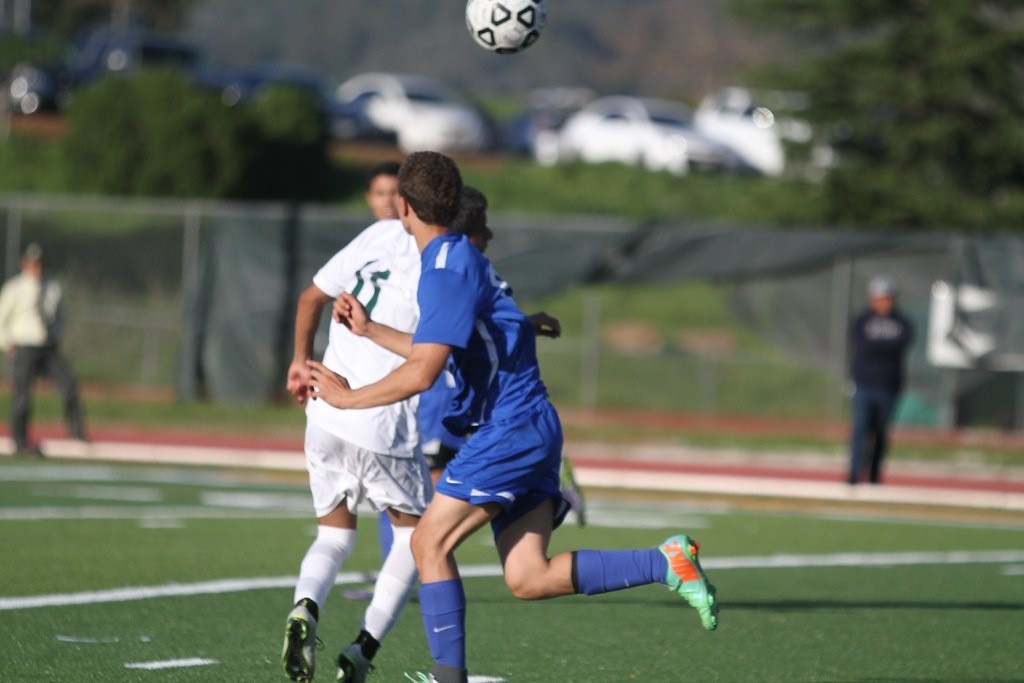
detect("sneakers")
[280,598,324,683]
[334,629,381,683]
[657,534,720,631]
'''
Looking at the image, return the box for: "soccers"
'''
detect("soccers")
[465,0,548,55]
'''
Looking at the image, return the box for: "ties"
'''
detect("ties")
[33,286,49,330]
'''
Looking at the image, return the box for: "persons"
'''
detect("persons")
[0,241,82,456]
[307,151,718,683]
[281,162,586,683]
[847,277,913,483]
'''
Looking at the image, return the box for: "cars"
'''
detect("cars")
[13,33,339,144]
[336,72,491,155]
[700,88,812,180]
[521,91,746,179]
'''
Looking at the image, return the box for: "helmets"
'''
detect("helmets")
[865,264,900,297]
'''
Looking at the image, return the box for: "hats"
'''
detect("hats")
[18,241,46,260]
